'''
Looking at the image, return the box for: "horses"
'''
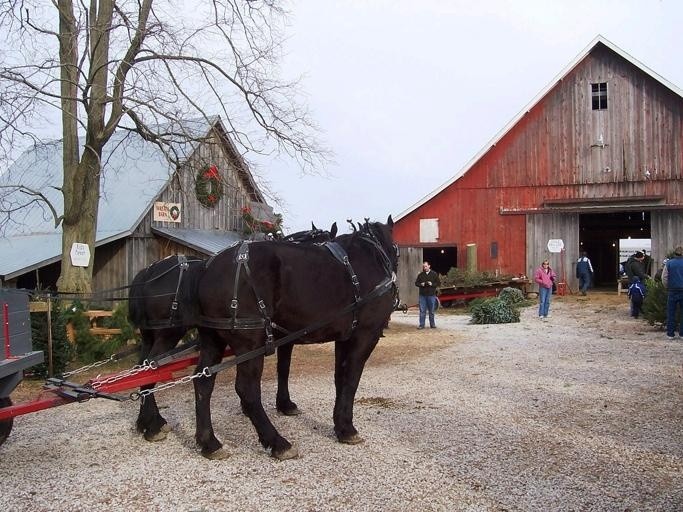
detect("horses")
[181,215,400,463]
[126,222,341,442]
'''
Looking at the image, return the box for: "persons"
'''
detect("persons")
[625,250,649,281]
[654,258,668,282]
[414,261,440,330]
[576,252,594,295]
[534,259,555,318]
[661,247,682,339]
[626,275,646,317]
